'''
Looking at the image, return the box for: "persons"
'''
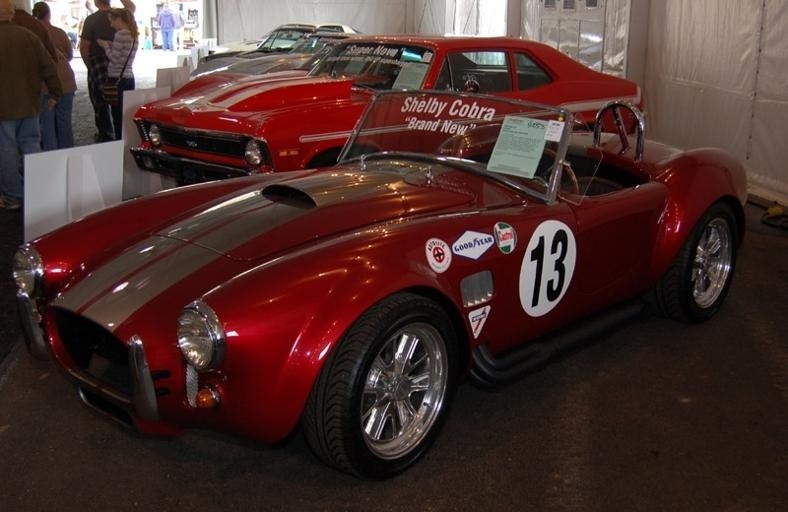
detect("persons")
[32,0,73,62]
[1,1,63,210]
[40,48,77,149]
[80,0,135,141]
[11,4,59,92]
[96,8,139,141]
[158,3,177,51]
[173,3,186,51]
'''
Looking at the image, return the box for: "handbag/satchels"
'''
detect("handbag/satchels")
[103,35,136,102]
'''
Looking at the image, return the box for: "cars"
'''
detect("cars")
[129,35,645,177]
[187,26,340,81]
[226,34,354,75]
[13,89,748,482]
[205,22,361,56]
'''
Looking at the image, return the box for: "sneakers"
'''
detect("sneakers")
[0,195,21,209]
